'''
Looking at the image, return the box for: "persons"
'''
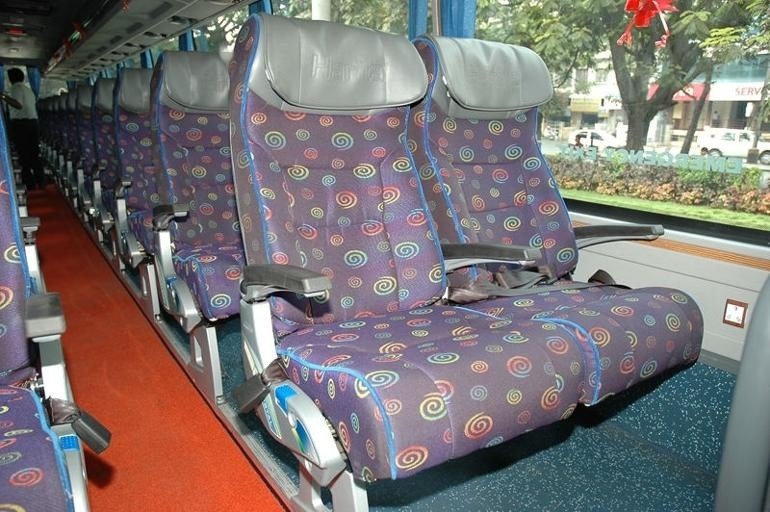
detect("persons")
[0,69,45,190]
[573,134,584,148]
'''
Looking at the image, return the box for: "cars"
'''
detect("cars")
[696,128,770,167]
[568,129,622,150]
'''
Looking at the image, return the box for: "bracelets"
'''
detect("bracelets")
[2,94,6,100]
